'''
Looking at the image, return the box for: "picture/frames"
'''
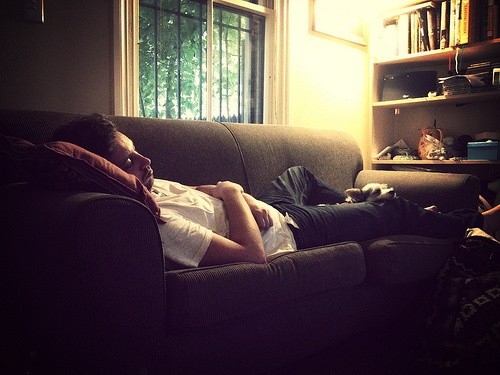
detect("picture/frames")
[309,0,370,52]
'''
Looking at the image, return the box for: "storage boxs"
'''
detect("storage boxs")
[467,141,499,160]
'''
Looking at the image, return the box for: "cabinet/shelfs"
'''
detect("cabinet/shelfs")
[369,0,500,237]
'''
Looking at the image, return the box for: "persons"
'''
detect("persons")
[54,112,500,268]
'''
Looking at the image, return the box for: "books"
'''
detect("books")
[384,0,494,56]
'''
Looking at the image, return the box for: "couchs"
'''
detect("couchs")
[0,108,485,375]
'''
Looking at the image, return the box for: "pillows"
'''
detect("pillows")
[0,134,168,224]
